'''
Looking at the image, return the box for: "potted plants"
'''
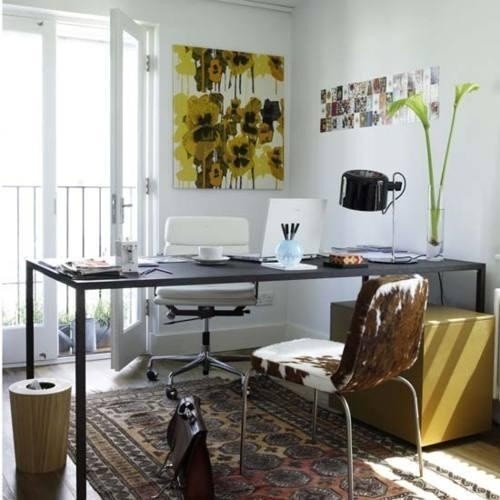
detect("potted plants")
[386,82,480,258]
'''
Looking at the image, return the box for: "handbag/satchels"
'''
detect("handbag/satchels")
[167,393,215,499]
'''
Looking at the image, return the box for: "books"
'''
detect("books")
[331,245,409,259]
[38,256,159,280]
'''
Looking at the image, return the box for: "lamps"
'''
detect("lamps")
[339,167,420,263]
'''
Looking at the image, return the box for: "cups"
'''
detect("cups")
[197,245,224,261]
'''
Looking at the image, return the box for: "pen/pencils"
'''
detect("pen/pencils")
[281,223,300,240]
[141,268,172,275]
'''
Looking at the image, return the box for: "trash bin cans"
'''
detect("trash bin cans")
[9,378,72,473]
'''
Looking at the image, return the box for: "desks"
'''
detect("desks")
[26,251,487,500]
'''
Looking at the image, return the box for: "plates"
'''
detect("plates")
[190,254,232,266]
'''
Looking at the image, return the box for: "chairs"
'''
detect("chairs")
[148,217,251,400]
[245,274,432,500]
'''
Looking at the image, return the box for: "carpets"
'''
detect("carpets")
[66,371,500,500]
